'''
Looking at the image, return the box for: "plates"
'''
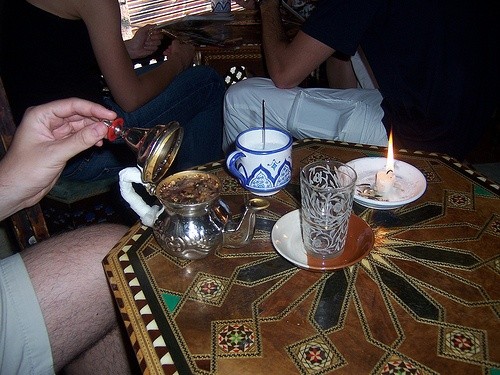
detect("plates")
[338,156,427,209]
[271,208,375,270]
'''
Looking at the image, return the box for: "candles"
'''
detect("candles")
[377,129,394,195]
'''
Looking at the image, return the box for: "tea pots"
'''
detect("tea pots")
[97,118,270,260]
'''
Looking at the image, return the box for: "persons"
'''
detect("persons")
[222,0,492,159]
[0,101,153,375]
[0,0,222,181]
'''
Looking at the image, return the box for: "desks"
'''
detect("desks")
[158,11,329,88]
[102,138,500,375]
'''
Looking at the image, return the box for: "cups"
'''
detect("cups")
[226,127,293,197]
[212,1,232,15]
[300,159,358,260]
